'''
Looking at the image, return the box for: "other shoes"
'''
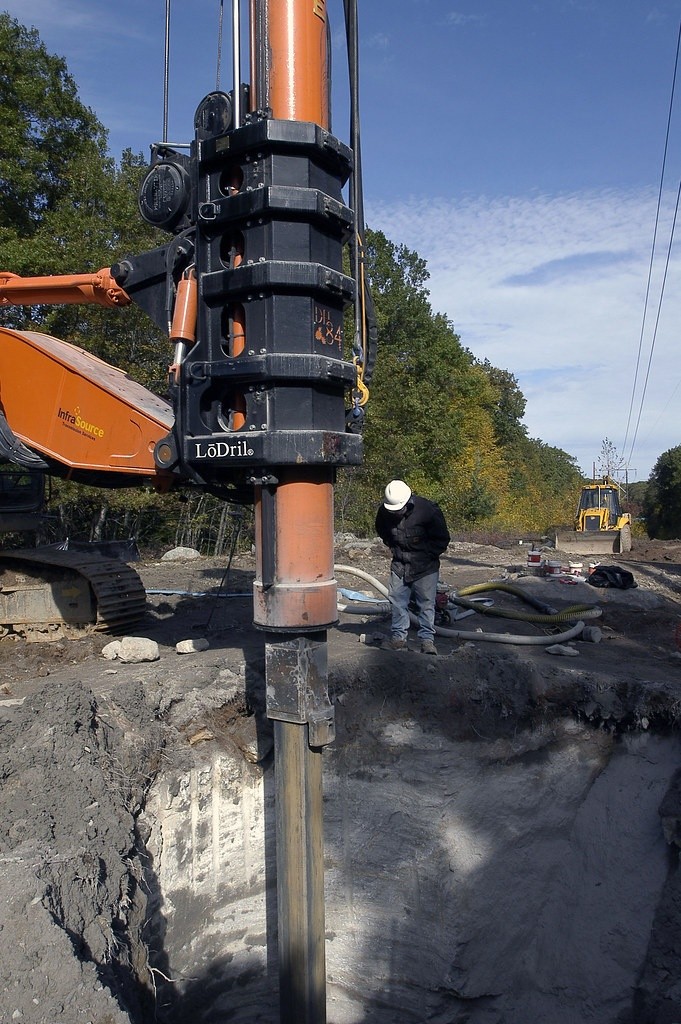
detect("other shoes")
[380,641,408,652]
[420,640,437,655]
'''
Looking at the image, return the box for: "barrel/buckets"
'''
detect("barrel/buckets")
[544,560,549,570]
[569,561,583,574]
[588,562,602,574]
[549,561,562,577]
[527,551,542,566]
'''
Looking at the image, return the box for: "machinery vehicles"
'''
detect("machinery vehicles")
[554,483,633,555]
[0,0,366,1023]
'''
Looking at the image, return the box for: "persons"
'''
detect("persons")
[374,478,450,654]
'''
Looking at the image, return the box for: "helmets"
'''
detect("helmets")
[384,480,411,510]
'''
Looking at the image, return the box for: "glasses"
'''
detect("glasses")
[388,506,405,513]
